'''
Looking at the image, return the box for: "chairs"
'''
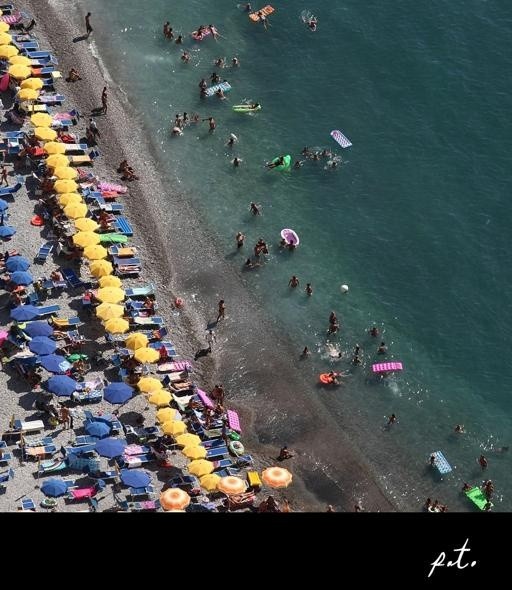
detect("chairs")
[0,0,262,513]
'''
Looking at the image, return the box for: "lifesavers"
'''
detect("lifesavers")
[230,440,245,454]
[272,155,292,171]
[301,9,315,22]
[320,373,332,384]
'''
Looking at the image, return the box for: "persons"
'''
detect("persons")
[1,1,500,513]
